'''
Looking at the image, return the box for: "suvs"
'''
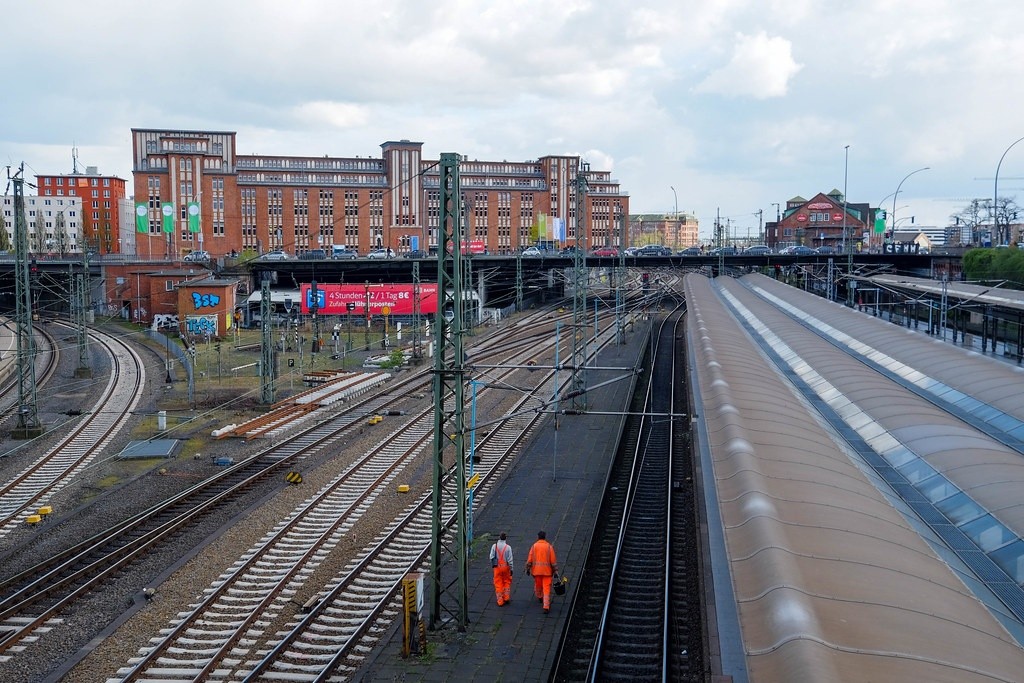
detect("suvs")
[632,245,666,257]
[298,249,327,260]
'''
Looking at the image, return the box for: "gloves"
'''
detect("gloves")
[526,570,530,576]
[554,574,558,579]
[510,571,513,576]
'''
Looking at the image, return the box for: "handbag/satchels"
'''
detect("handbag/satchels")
[492,542,497,568]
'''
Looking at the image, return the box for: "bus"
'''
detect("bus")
[447,241,485,255]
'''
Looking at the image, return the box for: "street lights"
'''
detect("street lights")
[878,166,931,241]
[191,191,203,247]
[671,185,678,223]
[59,200,88,260]
[841,145,849,255]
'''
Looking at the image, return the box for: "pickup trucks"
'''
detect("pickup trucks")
[252,311,288,327]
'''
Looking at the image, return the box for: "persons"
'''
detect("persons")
[490,533,513,607]
[527,532,559,614]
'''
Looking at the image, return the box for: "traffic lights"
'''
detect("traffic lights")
[31,259,37,272]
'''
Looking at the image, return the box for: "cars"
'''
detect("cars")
[706,246,737,256]
[367,248,397,259]
[677,247,703,256]
[624,247,639,256]
[743,245,772,255]
[592,247,618,257]
[778,245,835,255]
[522,247,541,256]
[260,251,289,261]
[331,250,358,260]
[918,248,929,255]
[183,250,210,261]
[665,248,672,255]
[403,249,428,259]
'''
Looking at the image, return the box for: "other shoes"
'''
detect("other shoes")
[539,598,543,602]
[543,609,548,614]
